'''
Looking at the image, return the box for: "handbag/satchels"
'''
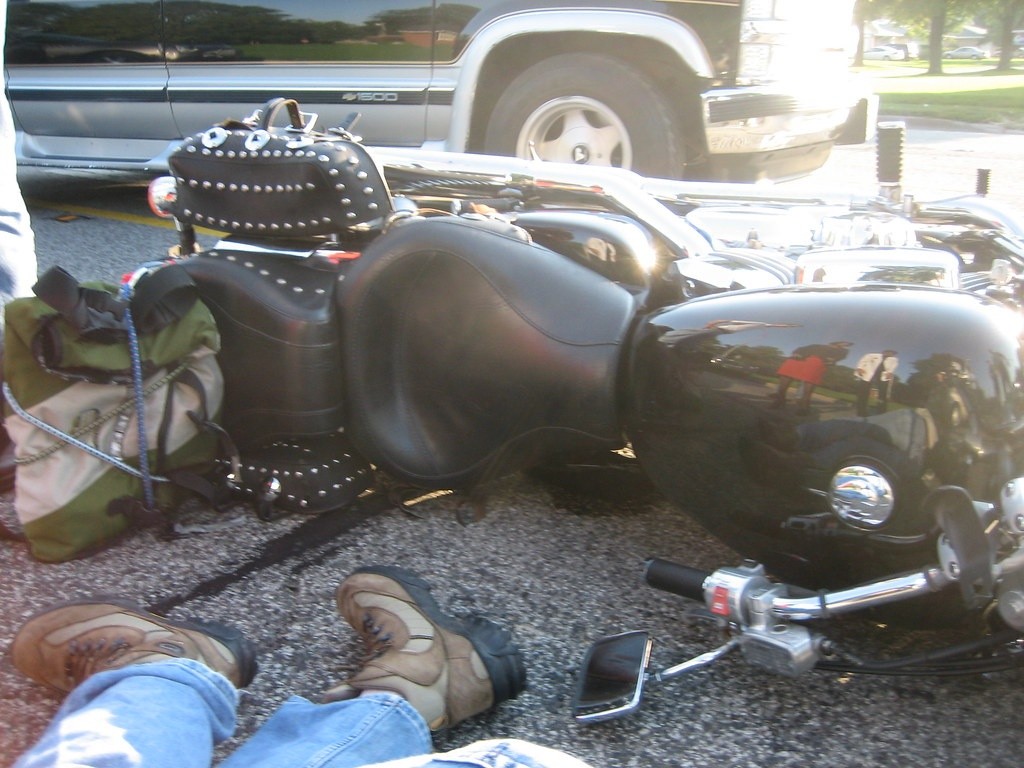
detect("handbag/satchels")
[2,264,223,565]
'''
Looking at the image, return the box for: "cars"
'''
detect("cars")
[7,2,863,201]
[942,47,986,61]
[864,45,904,62]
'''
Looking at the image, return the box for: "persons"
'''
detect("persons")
[9,563,593,767]
[0,0,36,334]
[854,349,899,415]
[740,381,979,468]
[660,319,803,349]
[774,341,854,414]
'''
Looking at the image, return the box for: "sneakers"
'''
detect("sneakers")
[325,564,526,732]
[10,598,254,697]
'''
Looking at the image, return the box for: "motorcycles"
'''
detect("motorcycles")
[120,97,1023,660]
